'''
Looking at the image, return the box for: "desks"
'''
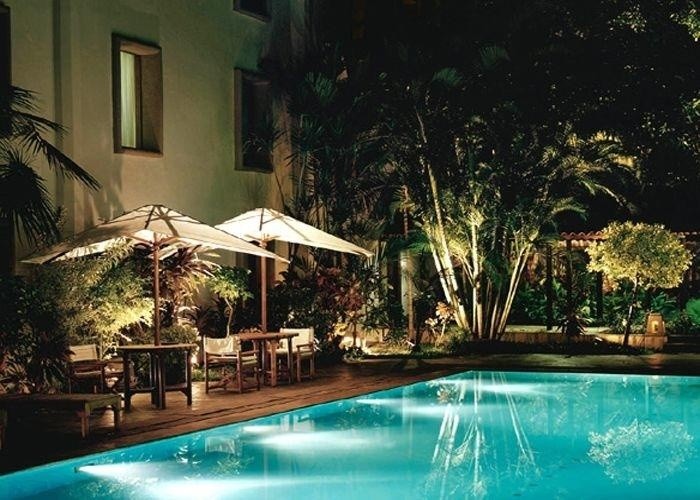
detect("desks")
[118,343,198,410]
[234,332,298,386]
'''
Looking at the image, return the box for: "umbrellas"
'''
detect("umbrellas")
[208,205,379,333]
[13,202,292,349]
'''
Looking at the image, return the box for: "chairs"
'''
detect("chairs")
[202,335,261,394]
[69,343,131,410]
[268,327,317,382]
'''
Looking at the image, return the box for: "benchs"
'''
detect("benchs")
[0,392,124,439]
